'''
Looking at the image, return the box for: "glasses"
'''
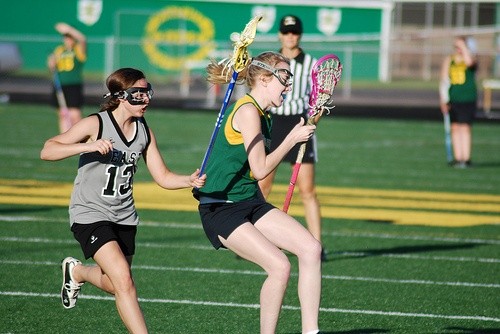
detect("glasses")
[252,61,294,85]
[105,83,153,105]
[282,31,299,35]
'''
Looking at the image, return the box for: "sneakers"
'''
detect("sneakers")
[60,257,84,310]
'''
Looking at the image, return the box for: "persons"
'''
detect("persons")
[191,50,325,334]
[40,68,208,334]
[255,14,321,243]
[46,23,87,134]
[439,35,479,167]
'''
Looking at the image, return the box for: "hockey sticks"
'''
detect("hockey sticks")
[439,78,454,163]
[51,65,72,136]
[283,55,343,213]
[191,12,267,191]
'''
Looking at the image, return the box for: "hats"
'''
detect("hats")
[279,15,302,32]
